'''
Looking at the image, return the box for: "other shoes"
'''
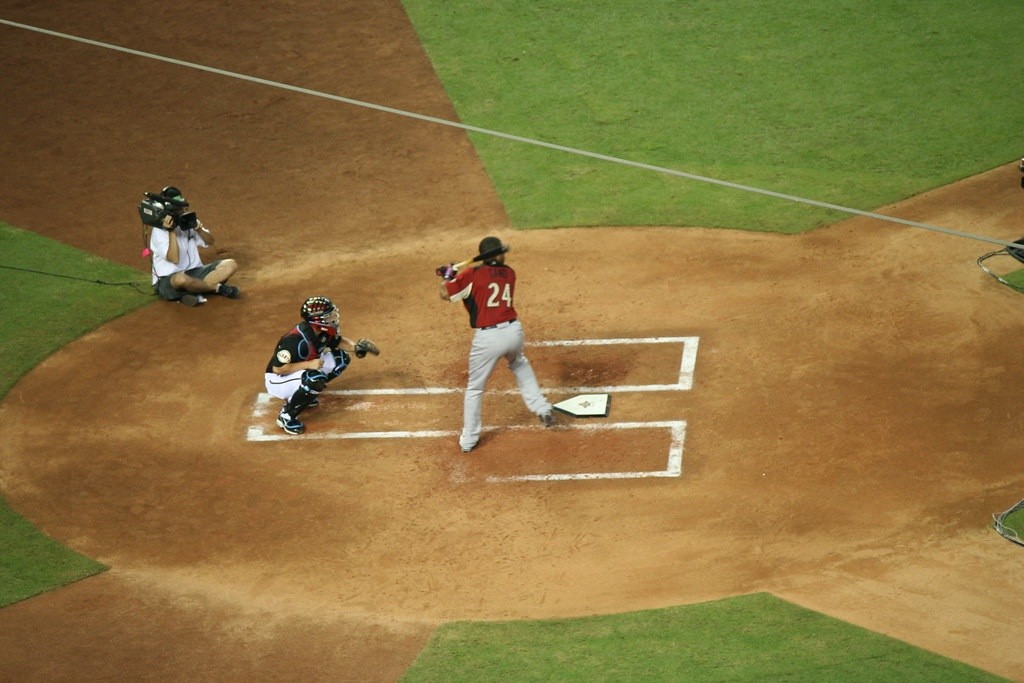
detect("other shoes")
[179,294,198,307]
[217,284,240,298]
[541,410,556,426]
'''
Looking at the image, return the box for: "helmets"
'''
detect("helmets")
[300,295,339,335]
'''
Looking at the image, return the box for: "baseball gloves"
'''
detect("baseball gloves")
[356,338,380,359]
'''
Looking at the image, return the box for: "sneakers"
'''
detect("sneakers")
[308,399,319,407]
[276,415,305,435]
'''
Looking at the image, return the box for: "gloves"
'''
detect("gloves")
[440,262,457,280]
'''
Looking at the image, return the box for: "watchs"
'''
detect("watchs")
[442,275,453,280]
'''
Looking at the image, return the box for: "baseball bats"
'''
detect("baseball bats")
[435,244,510,276]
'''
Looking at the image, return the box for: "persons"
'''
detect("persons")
[264,295,380,436]
[436,236,559,454]
[149,185,240,307]
